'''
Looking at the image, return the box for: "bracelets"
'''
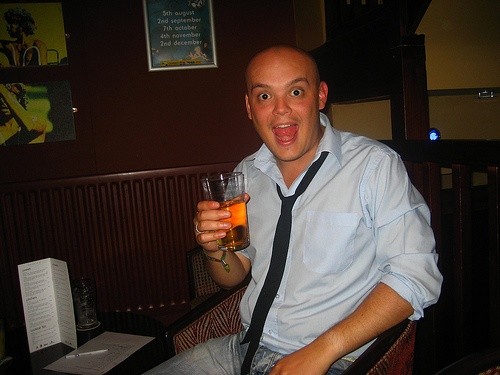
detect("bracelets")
[204,250,230,272]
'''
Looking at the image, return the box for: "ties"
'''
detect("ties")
[240,152,329,375]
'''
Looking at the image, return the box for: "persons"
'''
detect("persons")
[145,46,443,375]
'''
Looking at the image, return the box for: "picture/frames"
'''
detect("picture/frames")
[143,0,218,73]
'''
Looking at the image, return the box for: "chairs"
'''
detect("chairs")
[186,247,222,310]
[162,280,416,375]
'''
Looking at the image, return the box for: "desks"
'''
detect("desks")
[0,310,169,375]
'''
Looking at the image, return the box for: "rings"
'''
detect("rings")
[196,224,204,234]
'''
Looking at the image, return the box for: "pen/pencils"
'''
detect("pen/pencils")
[65,349,110,358]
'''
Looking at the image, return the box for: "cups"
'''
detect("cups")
[72,278,98,330]
[202,172,251,252]
